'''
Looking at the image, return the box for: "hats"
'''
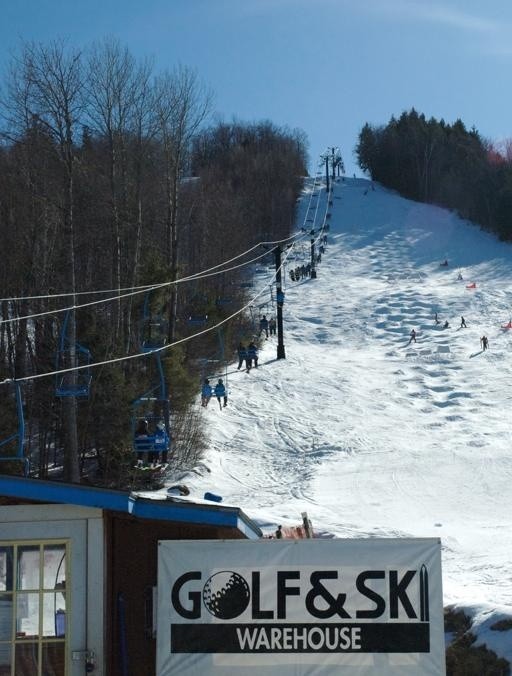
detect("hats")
[218,379,223,383]
[205,378,209,384]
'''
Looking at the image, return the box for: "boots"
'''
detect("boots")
[134,460,169,473]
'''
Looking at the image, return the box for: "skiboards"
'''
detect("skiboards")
[134,462,169,474]
[202,402,228,411]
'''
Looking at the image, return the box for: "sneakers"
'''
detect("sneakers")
[201,403,208,407]
[220,404,228,411]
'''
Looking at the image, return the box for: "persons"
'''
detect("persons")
[408,329,416,343]
[134,418,170,471]
[275,525,283,539]
[459,316,466,328]
[434,312,439,324]
[258,315,278,338]
[439,259,448,266]
[480,336,488,351]
[199,379,230,412]
[288,223,329,281]
[443,320,448,327]
[235,343,260,372]
